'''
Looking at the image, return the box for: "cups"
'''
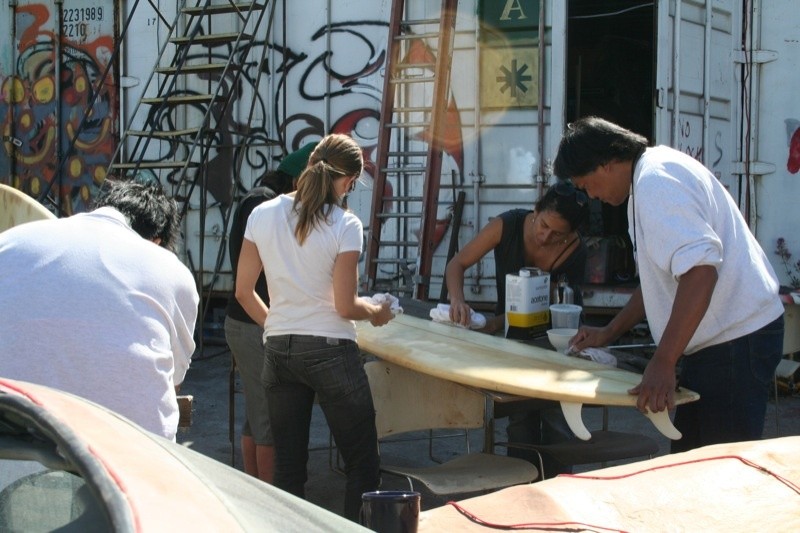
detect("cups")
[549,304,582,329]
[359,490,421,533]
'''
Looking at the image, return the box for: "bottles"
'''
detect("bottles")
[553,271,574,304]
[504,266,551,347]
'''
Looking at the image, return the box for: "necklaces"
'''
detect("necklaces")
[627,142,644,278]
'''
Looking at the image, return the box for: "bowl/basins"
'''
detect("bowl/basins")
[546,328,578,355]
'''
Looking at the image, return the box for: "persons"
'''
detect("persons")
[445,182,589,484]
[224,140,319,490]
[234,133,395,524]
[551,117,785,458]
[0,173,201,448]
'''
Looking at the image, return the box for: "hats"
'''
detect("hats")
[277,141,321,175]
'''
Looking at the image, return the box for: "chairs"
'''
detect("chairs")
[365,362,661,509]
